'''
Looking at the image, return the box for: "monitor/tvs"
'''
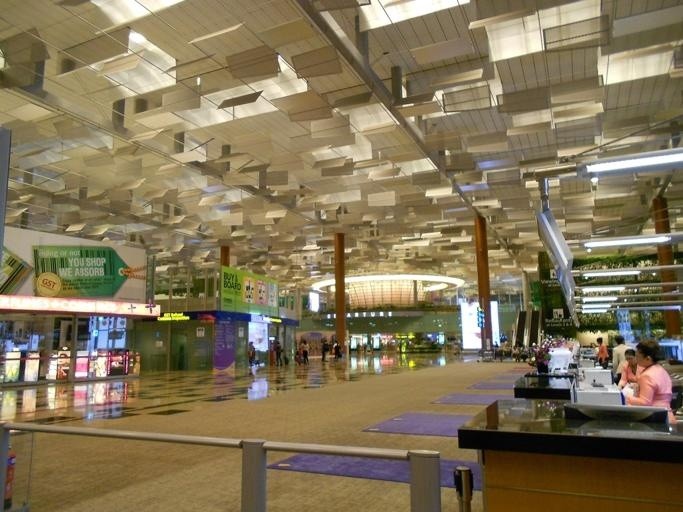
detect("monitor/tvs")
[569,378,577,404]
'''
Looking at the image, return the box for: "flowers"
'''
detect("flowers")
[531,334,574,373]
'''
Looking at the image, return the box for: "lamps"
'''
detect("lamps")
[556,113,683,180]
[574,199,683,315]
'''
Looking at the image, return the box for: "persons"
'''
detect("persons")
[591,334,677,426]
[512,342,530,363]
[245,335,343,366]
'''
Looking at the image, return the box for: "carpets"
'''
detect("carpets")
[266,367,539,492]
